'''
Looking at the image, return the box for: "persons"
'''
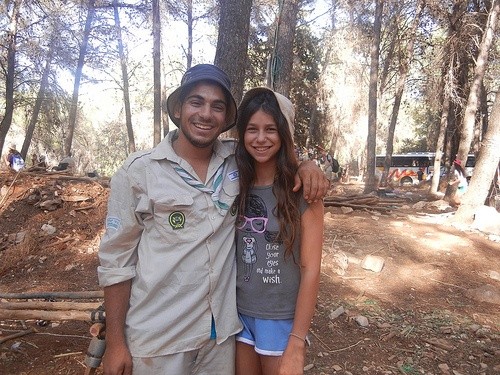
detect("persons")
[411,168,432,186]
[4,144,74,173]
[292,141,348,182]
[96,64,329,375]
[448,161,468,209]
[235,87,324,375]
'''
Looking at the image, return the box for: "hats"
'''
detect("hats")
[237,87,295,147]
[167,64,237,133]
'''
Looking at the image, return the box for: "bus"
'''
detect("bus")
[375,152,475,187]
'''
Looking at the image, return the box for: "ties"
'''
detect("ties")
[169,158,230,217]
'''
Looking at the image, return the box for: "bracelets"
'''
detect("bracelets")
[288,333,306,345]
